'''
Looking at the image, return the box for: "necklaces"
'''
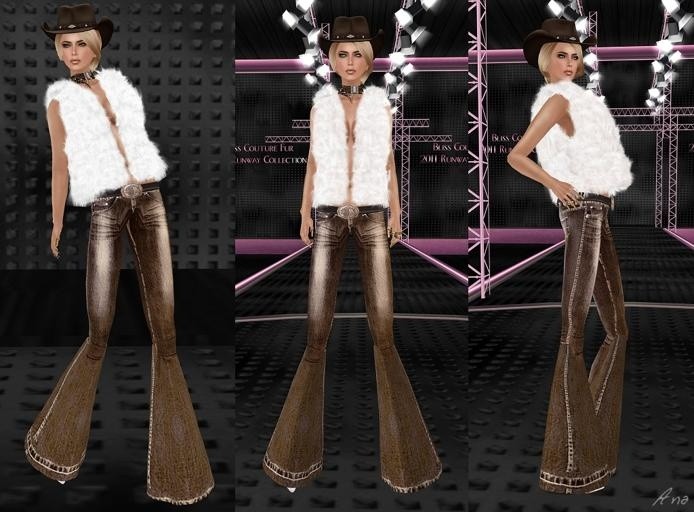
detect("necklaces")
[70,70,99,88]
[338,84,367,102]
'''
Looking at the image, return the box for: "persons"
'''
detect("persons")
[20,4,220,507]
[257,15,444,495]
[502,18,638,495]
